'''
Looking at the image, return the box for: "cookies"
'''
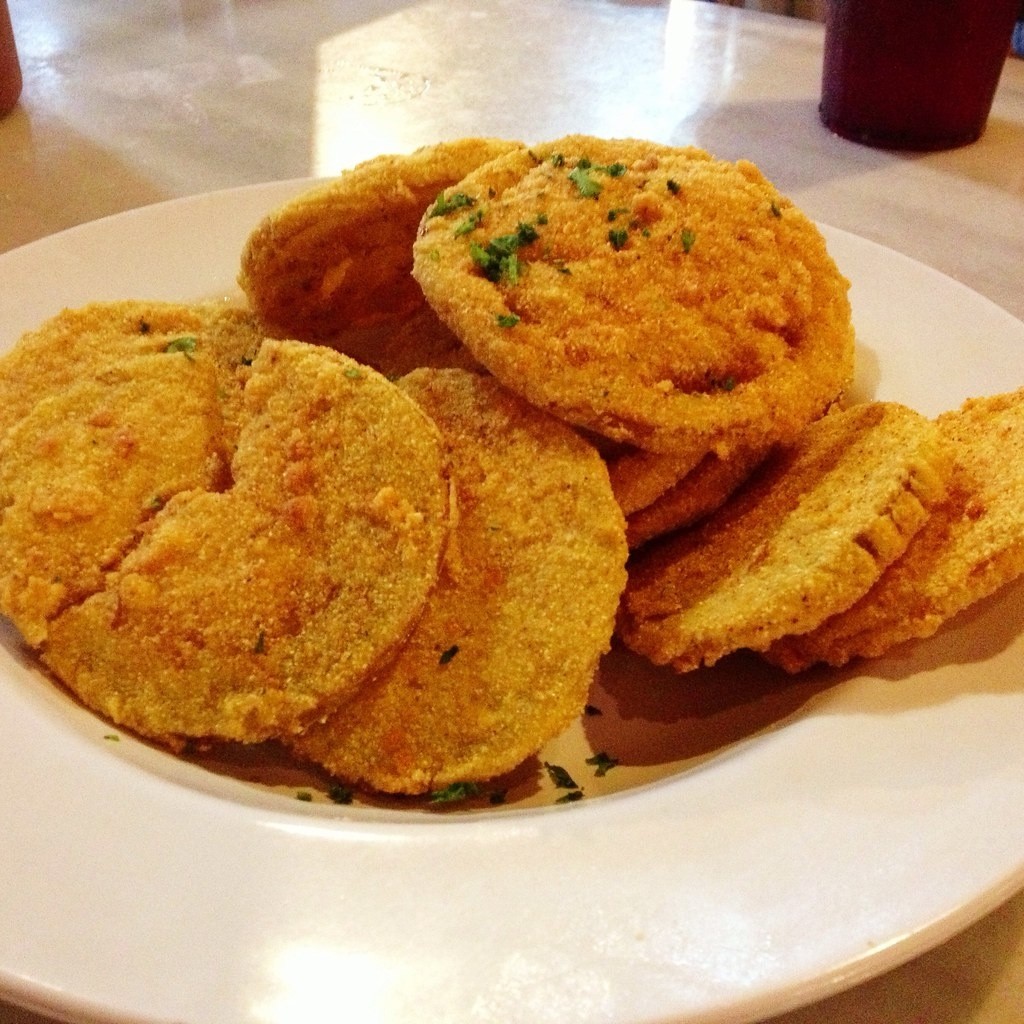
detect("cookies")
[0,136,1024,796]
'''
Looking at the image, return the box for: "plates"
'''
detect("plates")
[0,173,1024,1024]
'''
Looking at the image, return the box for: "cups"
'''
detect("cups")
[819,0,1024,155]
[0,0,24,120]
